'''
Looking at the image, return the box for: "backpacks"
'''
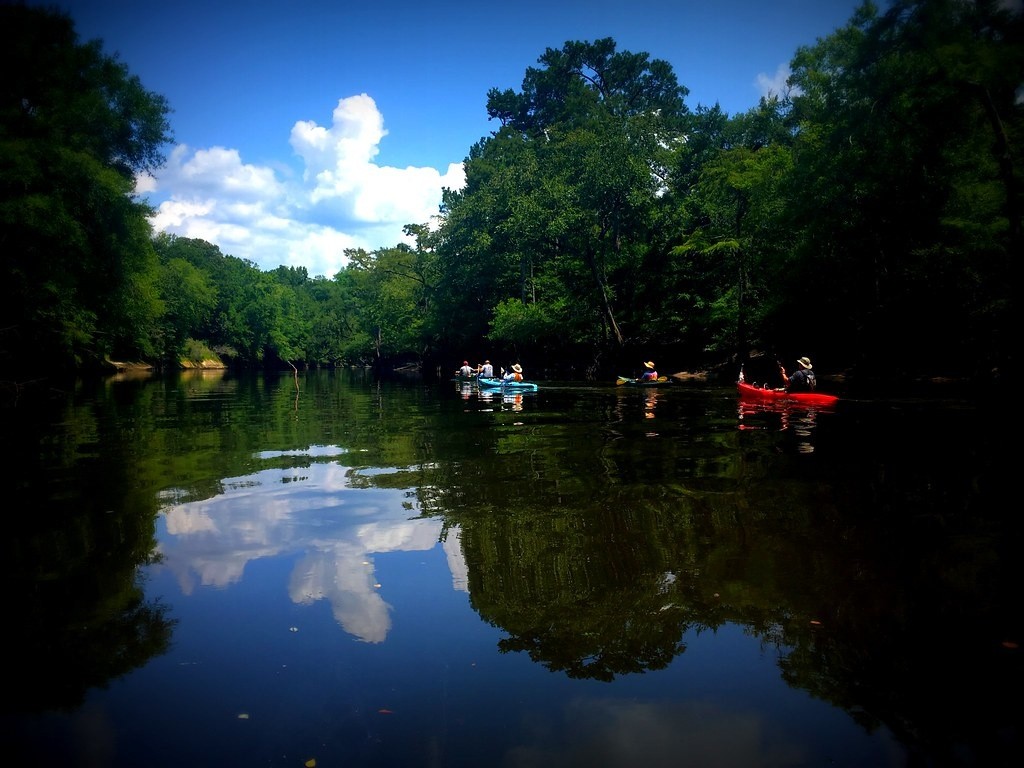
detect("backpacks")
[800,370,816,393]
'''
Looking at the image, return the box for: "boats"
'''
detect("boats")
[733,380,839,409]
[479,378,537,389]
[617,376,676,385]
[455,371,481,381]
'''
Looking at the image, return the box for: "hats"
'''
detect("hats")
[797,357,812,369]
[644,361,655,369]
[511,364,522,372]
[464,361,468,365]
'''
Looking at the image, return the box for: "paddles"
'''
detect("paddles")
[616,376,667,385]
[501,367,511,375]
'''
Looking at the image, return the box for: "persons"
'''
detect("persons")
[493,363,523,383]
[753,357,817,394]
[636,361,658,382]
[457,361,474,378]
[479,360,493,378]
